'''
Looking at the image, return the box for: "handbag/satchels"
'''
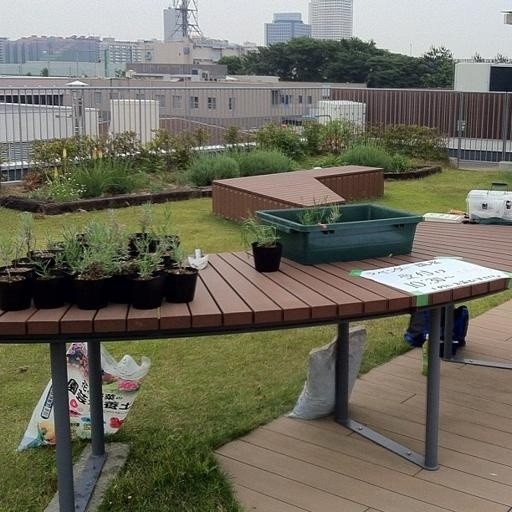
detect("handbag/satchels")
[466,183,512,224]
[406,306,468,356]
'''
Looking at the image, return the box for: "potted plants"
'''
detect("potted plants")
[2,213,201,312]
[260,203,424,266]
[237,214,285,274]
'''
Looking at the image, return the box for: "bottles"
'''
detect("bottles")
[420,333,432,377]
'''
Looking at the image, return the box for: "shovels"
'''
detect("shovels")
[187,249,209,270]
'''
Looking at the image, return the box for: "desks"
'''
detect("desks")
[0,213,512,511]
